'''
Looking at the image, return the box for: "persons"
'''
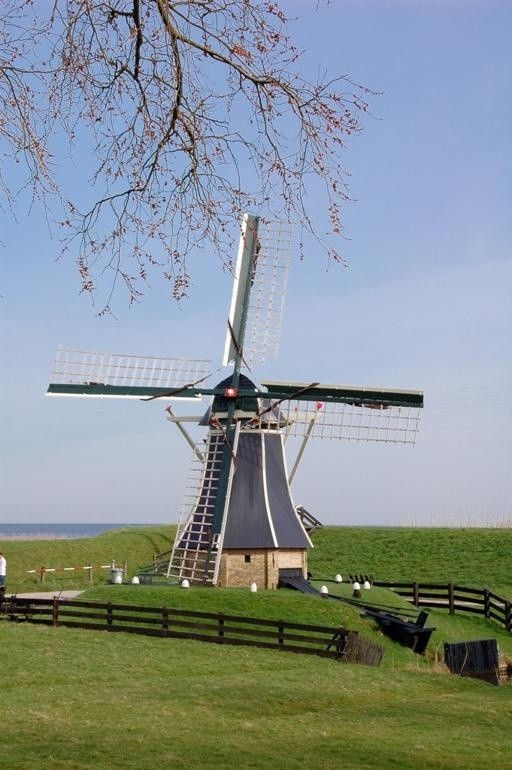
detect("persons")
[0,552,6,597]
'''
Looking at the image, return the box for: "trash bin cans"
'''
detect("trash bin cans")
[112,570,123,585]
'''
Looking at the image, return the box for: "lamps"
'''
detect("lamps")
[364,581,370,590]
[130,576,140,585]
[352,582,360,590]
[180,579,190,588]
[250,582,257,593]
[335,574,342,584]
[320,586,329,594]
